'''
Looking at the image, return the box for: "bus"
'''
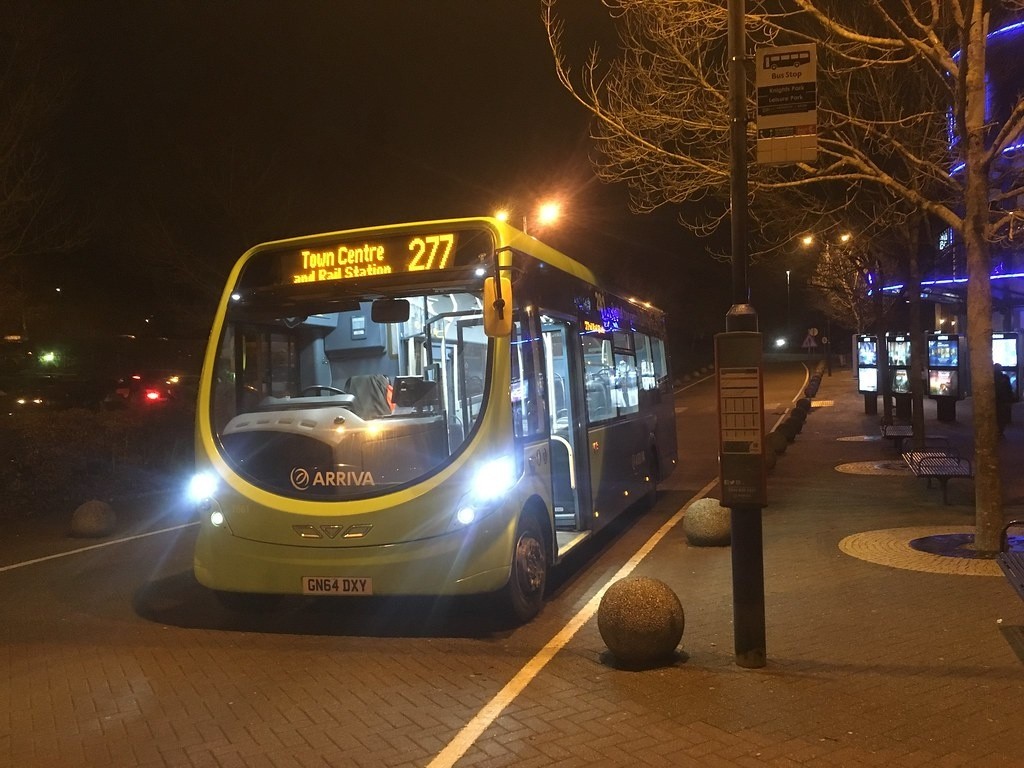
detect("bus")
[191,216,679,627]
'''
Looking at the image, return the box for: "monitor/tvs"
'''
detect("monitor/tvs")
[391,375,424,404]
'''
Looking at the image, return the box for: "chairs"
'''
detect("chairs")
[586,379,611,420]
[345,374,391,420]
[553,372,567,420]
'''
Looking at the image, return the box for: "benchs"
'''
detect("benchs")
[901,434,975,506]
[996,519,1024,603]
[878,414,915,453]
